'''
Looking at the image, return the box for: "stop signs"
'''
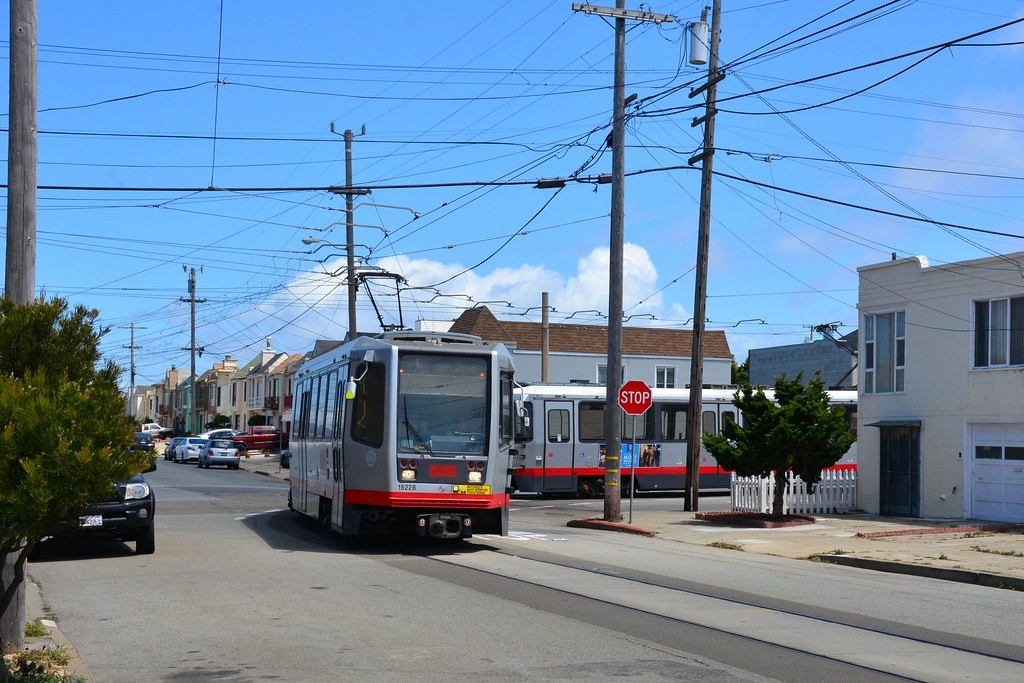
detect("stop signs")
[618,380,652,415]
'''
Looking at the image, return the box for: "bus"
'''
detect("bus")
[509,378,859,500]
[287,330,515,541]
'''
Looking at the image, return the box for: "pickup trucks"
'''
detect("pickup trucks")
[221,426,289,456]
[142,420,174,440]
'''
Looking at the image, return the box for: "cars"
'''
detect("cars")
[208,431,248,440]
[164,437,185,460]
[174,438,210,464]
[199,429,236,439]
[130,432,156,451]
[198,439,240,471]
[28,471,156,559]
